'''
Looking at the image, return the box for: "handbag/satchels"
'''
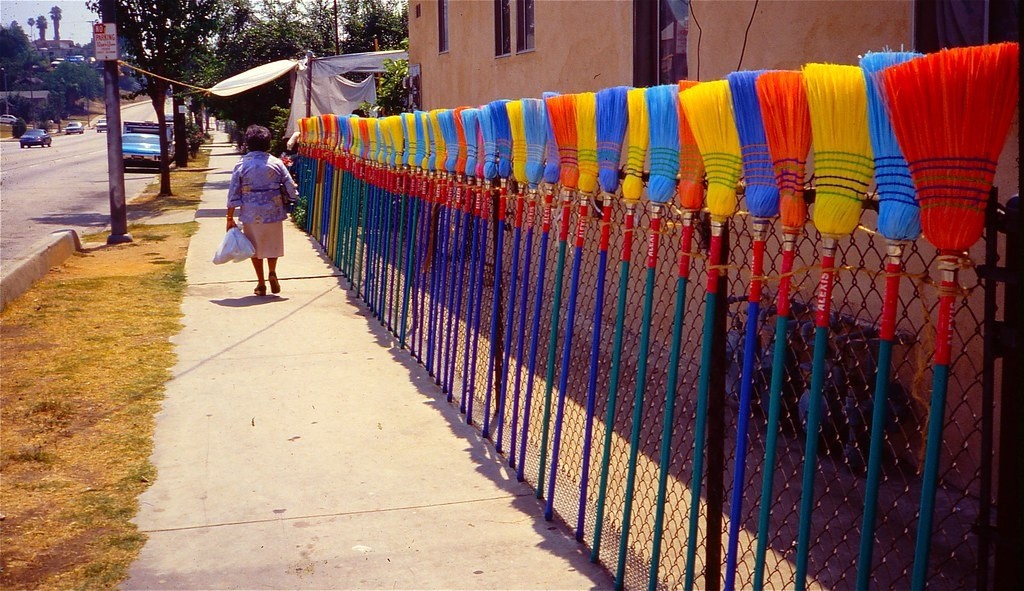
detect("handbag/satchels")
[280,183,290,205]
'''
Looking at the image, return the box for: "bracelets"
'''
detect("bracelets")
[226,215,232,218]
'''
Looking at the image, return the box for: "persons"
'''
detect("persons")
[226,124,300,295]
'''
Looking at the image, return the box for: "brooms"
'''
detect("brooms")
[289,36,1024,591]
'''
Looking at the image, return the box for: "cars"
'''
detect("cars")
[96,118,107,133]
[19,128,52,148]
[122,112,175,173]
[0,114,18,125]
[65,122,84,134]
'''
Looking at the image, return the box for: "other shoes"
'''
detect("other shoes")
[268,271,281,293]
[254,284,267,296]
